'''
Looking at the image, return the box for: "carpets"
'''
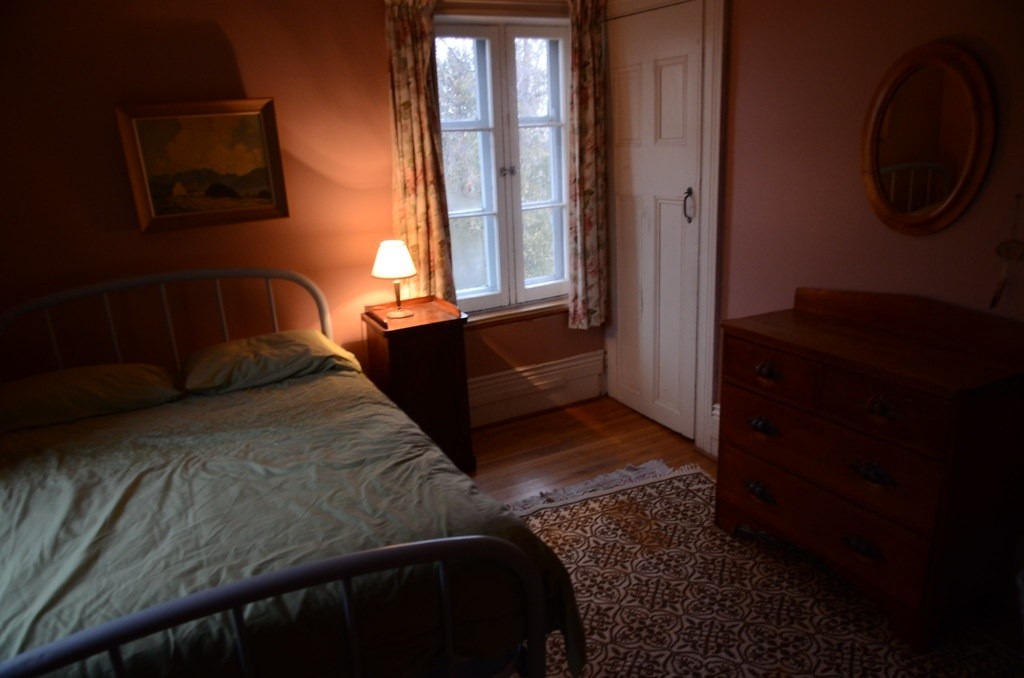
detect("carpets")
[505,457,916,678]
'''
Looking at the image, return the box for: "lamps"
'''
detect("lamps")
[372,237,417,318]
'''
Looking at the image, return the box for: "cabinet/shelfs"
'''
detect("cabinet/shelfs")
[716,289,1024,678]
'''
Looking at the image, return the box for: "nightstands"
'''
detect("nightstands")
[362,296,478,479]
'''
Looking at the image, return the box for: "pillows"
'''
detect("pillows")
[0,365,176,431]
[186,328,362,395]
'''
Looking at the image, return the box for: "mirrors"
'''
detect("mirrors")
[861,38,997,233]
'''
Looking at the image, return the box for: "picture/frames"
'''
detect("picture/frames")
[115,96,290,235]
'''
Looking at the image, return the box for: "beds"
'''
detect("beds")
[0,265,585,677]
